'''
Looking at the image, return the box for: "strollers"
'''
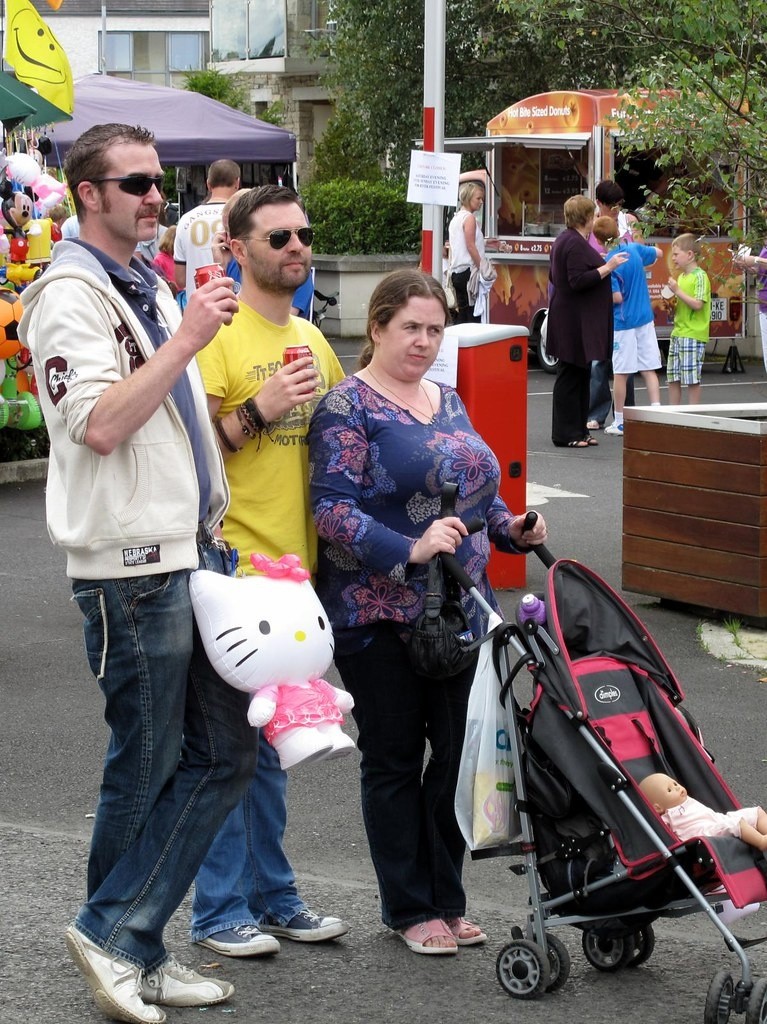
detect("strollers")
[446,514,767,1023]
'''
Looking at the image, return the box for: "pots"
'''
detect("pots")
[525,223,549,235]
[549,223,567,236]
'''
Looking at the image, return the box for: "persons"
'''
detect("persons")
[545,180,662,448]
[18,122,261,1024]
[304,266,547,956]
[189,184,351,962]
[727,242,767,373]
[51,160,314,320]
[660,233,712,404]
[419,182,498,325]
[637,772,767,851]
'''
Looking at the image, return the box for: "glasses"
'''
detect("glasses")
[237,226,315,248]
[607,198,626,211]
[70,173,163,196]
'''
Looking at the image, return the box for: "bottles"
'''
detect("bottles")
[518,593,546,629]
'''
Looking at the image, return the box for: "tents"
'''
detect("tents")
[0,72,74,137]
[10,72,298,225]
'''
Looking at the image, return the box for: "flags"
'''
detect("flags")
[7,0,74,115]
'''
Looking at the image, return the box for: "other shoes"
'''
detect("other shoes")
[586,420,599,430]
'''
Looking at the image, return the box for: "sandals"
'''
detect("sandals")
[567,435,598,447]
[394,915,487,955]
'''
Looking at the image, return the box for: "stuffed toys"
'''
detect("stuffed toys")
[188,552,359,770]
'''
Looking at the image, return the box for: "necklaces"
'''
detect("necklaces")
[366,364,434,419]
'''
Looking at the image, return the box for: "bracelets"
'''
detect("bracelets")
[215,418,238,453]
[236,398,276,452]
[753,256,760,265]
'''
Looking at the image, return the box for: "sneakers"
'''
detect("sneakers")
[604,421,624,436]
[63,925,168,1024]
[195,924,280,957]
[137,954,236,1007]
[257,909,351,942]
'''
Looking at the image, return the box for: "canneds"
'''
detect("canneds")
[283,345,314,394]
[194,264,230,300]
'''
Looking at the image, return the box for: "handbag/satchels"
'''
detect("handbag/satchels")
[407,481,479,680]
[452,611,522,851]
[442,284,458,308]
